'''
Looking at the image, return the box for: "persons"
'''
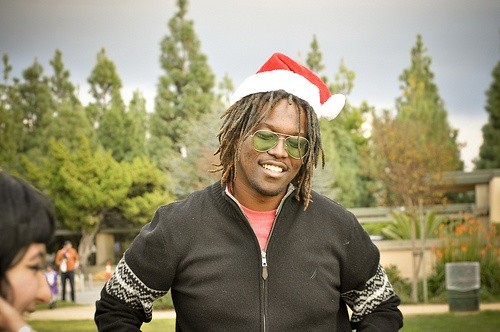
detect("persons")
[0,172,53,332]
[44,264,59,304]
[55,240,79,303]
[94,53,404,332]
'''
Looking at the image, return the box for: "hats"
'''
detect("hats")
[231,52,345,121]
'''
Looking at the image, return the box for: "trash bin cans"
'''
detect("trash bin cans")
[445,261,480,312]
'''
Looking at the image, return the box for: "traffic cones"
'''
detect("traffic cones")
[104,261,112,282]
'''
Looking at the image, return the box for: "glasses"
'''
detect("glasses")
[247,130,311,160]
